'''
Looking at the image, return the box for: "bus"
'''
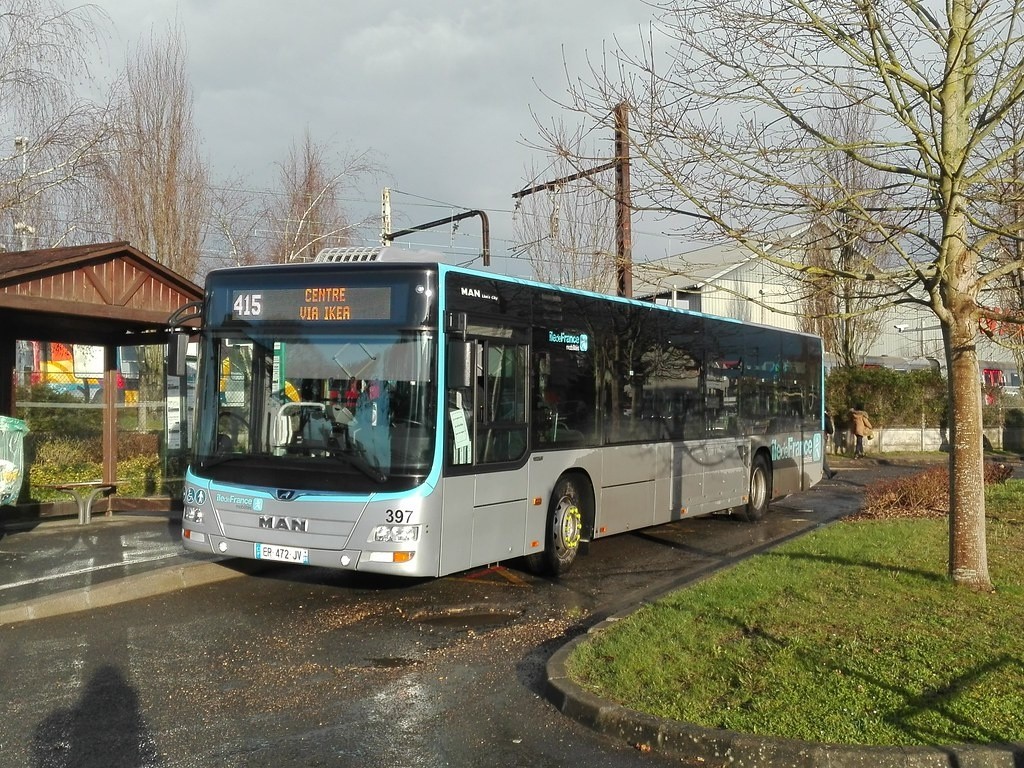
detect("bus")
[161,240,831,583]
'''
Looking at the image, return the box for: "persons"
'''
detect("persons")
[263,324,825,479]
[823,406,839,480]
[847,403,873,460]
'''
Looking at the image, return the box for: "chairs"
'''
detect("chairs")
[541,410,797,446]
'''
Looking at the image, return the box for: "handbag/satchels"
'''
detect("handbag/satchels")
[867,434,874,440]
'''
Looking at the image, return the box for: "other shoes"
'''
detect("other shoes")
[828,470,838,479]
[854,452,866,458]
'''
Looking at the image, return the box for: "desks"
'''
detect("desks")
[31,480,129,526]
